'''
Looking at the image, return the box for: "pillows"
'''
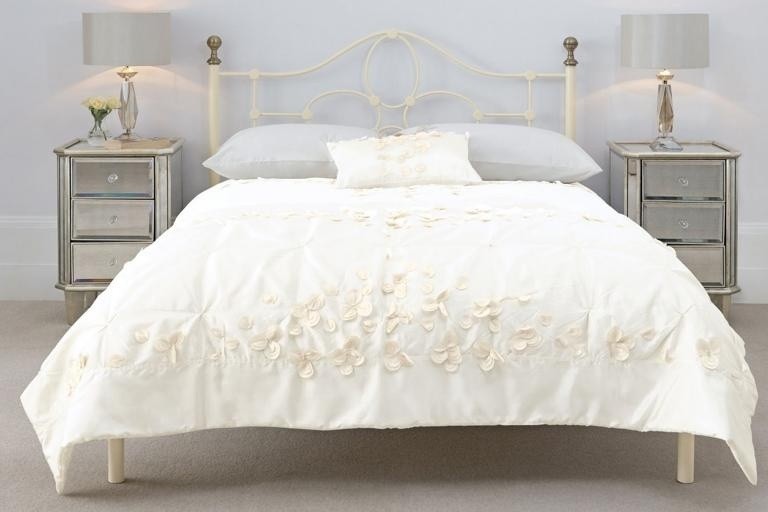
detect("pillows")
[326,126,487,191]
[431,119,604,186]
[200,120,340,179]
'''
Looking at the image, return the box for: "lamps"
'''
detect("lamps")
[77,11,174,142]
[615,12,711,155]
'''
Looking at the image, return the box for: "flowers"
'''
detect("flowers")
[82,94,123,140]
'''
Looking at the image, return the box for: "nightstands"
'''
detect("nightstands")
[601,139,742,326]
[53,140,184,326]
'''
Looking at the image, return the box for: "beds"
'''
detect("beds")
[11,30,760,503]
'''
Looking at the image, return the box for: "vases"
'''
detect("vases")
[85,118,108,146]
